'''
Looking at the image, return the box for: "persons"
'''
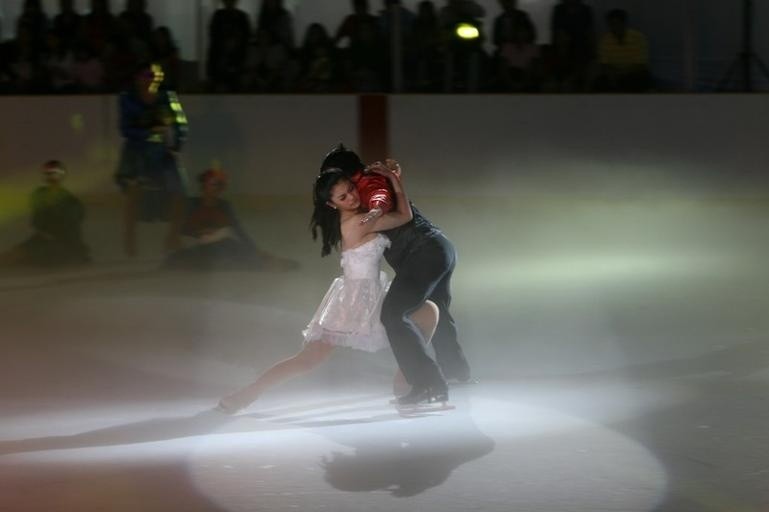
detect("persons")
[159,167,297,275]
[317,141,473,408]
[2,2,651,95]
[319,416,499,498]
[209,165,440,419]
[113,61,190,263]
[1,156,99,269]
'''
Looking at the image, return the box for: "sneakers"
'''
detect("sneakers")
[394,383,449,407]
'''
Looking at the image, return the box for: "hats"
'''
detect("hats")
[199,170,227,193]
